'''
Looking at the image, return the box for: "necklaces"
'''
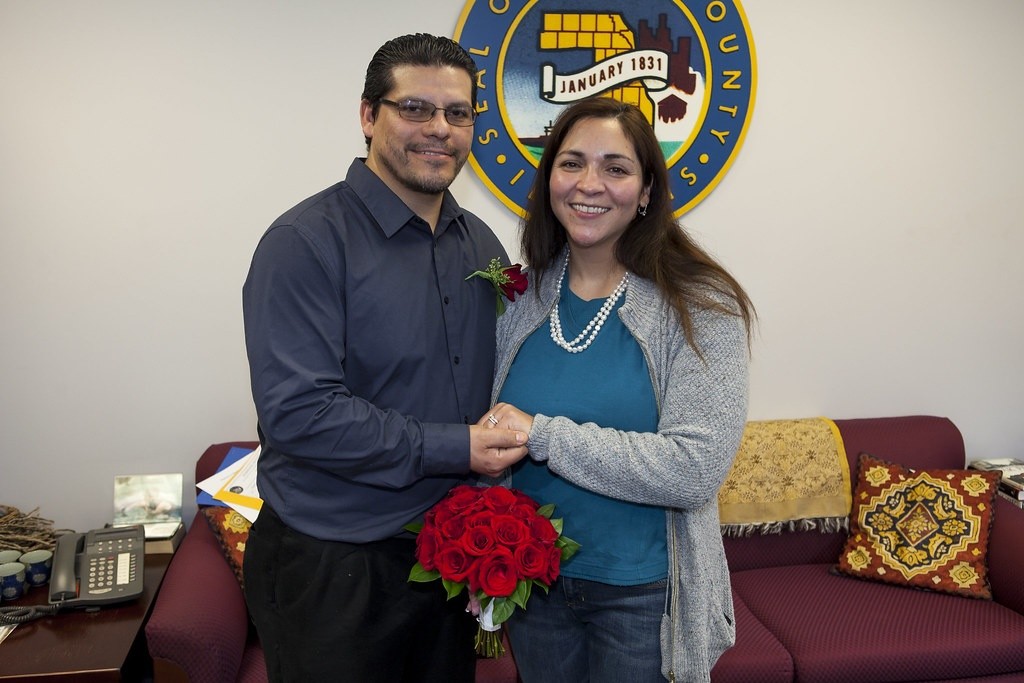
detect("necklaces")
[549,251,629,353]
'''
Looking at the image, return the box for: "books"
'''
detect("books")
[967,457,1024,509]
[110,522,186,554]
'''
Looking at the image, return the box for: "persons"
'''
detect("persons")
[478,98,753,683]
[242,33,528,683]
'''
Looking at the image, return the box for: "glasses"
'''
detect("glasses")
[380,97,480,127]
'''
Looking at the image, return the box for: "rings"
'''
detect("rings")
[489,416,498,426]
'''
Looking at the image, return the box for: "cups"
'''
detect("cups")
[0,549,23,565]
[19,549,53,587]
[0,562,26,601]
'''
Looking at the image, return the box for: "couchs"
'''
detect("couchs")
[146,415,1023,682]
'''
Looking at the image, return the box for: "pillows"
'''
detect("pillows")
[827,451,1001,601]
[200,505,254,591]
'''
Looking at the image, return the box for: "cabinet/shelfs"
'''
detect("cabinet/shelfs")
[0,529,186,682]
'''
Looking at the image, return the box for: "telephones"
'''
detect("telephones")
[45,524,146,609]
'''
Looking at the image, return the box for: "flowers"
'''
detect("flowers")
[464,256,528,317]
[401,485,582,659]
